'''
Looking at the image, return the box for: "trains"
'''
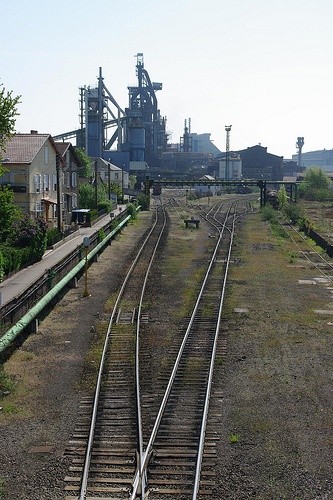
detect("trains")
[153,185,161,195]
